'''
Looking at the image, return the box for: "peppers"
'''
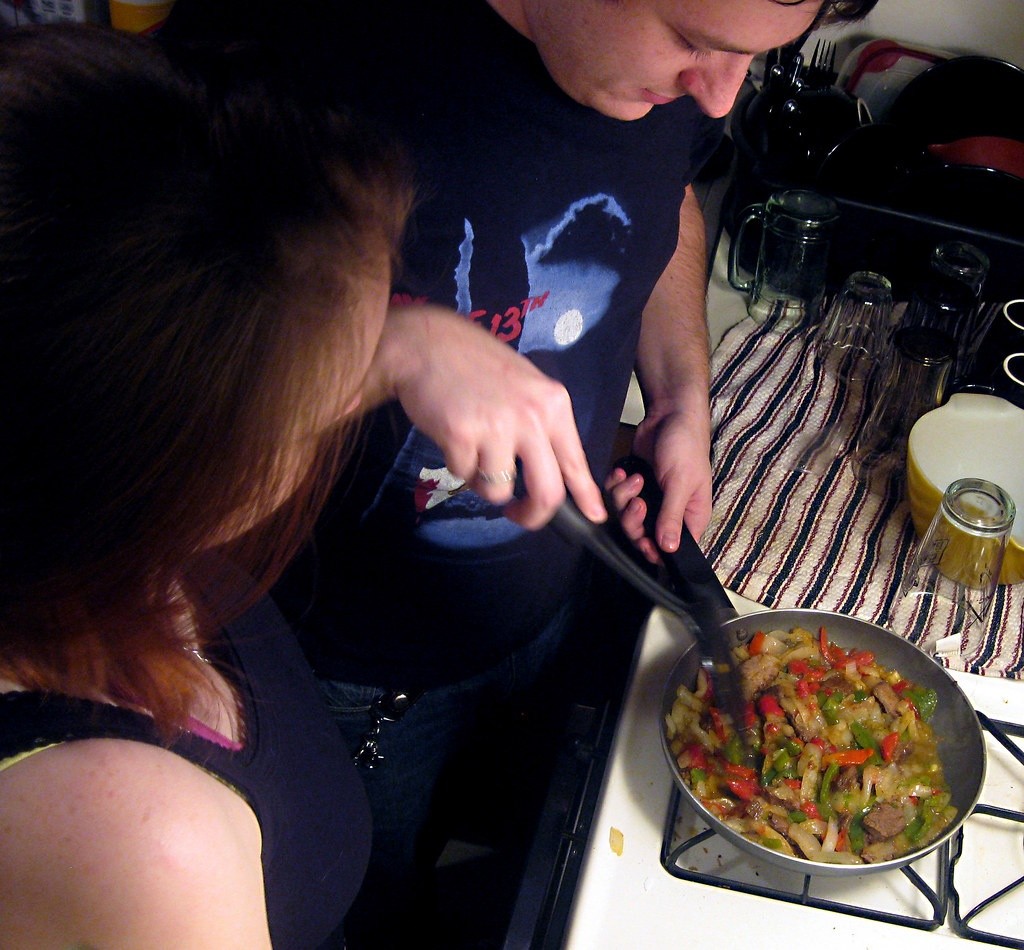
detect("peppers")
[681,624,939,851]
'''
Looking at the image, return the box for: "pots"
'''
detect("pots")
[612,455,988,878]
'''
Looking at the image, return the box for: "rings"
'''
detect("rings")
[478,469,515,482]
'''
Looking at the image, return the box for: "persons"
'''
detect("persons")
[0,0,882,949]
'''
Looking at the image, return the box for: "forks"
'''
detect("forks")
[806,38,837,92]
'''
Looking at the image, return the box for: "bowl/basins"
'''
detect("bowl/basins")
[908,392,1024,586]
[818,55,1024,241]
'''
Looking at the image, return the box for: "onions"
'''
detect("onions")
[665,631,931,866]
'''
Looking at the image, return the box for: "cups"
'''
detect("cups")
[890,478,1016,657]
[851,241,991,498]
[727,188,838,333]
[724,89,856,239]
[819,270,894,381]
